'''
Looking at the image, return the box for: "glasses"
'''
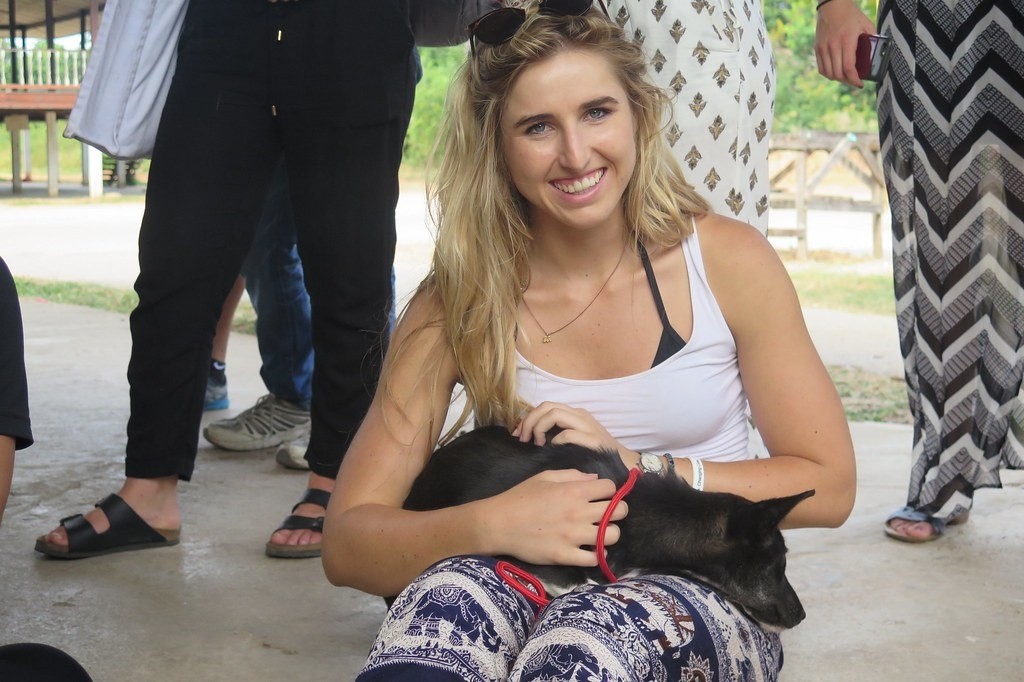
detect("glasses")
[467,0,594,59]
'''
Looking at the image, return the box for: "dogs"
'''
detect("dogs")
[383,425,815,632]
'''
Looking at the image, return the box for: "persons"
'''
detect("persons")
[0,255,91,682]
[817,0,1024,540]
[33,0,856,680]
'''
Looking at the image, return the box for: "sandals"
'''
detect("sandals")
[884,506,969,543]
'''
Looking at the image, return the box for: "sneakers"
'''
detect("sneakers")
[202,391,311,451]
[202,377,229,411]
[275,433,311,470]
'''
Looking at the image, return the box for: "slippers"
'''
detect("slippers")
[34,492,182,559]
[264,488,332,559]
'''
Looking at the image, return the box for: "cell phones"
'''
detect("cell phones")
[856,33,892,82]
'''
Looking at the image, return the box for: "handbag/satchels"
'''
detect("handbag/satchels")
[62,0,190,159]
